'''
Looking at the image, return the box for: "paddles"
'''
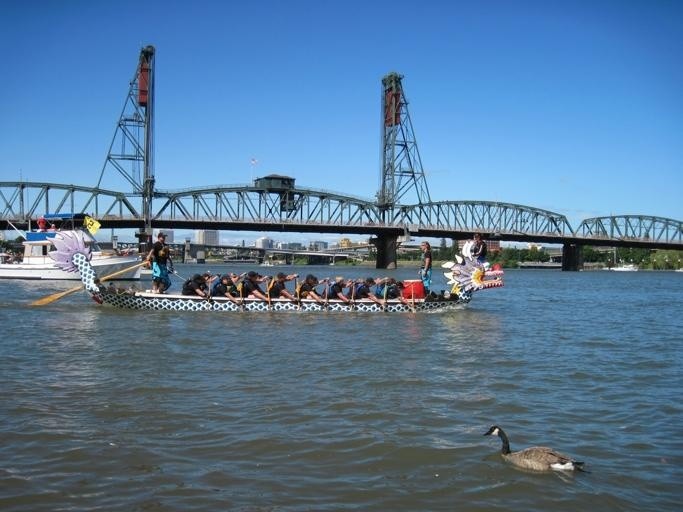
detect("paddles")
[32,261,147,305]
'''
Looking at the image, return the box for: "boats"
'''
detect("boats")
[0,216,142,281]
[45,230,504,314]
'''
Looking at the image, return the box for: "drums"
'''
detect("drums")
[403,279,426,299]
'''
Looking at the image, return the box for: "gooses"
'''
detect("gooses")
[485,424,586,474]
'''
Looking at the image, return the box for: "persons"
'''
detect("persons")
[417,241,432,294]
[472,233,488,262]
[182,271,414,307]
[146,232,173,293]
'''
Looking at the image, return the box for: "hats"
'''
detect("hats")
[158,233,167,236]
[193,271,405,289]
